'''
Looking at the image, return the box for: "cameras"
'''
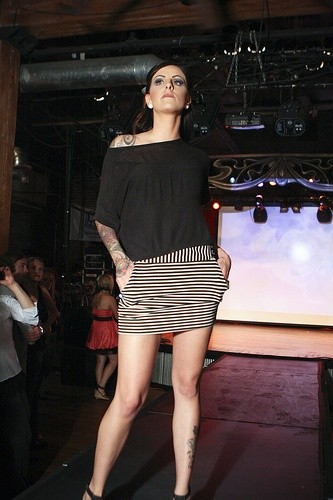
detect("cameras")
[0,271,4,280]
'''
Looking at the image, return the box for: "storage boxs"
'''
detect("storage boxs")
[81,254,105,285]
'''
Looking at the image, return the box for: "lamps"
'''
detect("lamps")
[291,202,302,213]
[280,201,288,213]
[253,191,268,223]
[317,196,333,224]
